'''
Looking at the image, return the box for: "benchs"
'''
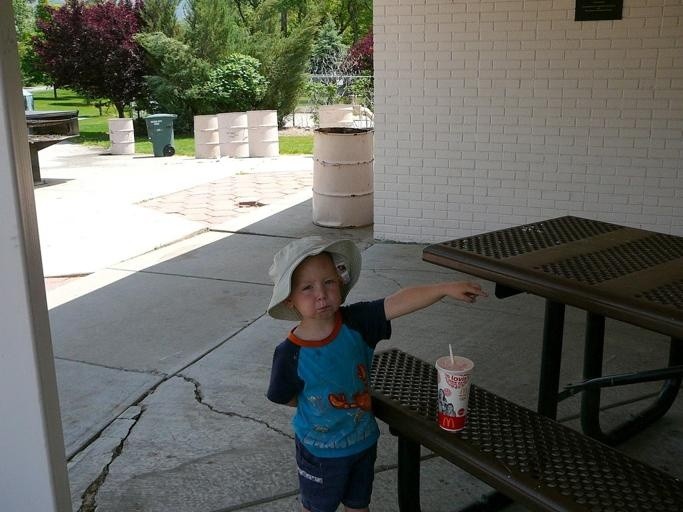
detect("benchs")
[369,348,683,511]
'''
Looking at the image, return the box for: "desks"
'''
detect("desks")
[420,214,683,446]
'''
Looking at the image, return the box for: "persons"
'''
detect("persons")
[263,233,492,512]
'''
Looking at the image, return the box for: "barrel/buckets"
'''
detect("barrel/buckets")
[319,103,374,129]
[312,127,375,229]
[106,118,135,155]
[216,112,249,158]
[247,109,279,158]
[193,115,219,159]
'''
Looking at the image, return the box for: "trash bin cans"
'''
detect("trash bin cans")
[143,114,177,157]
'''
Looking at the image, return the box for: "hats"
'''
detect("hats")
[268,236,361,322]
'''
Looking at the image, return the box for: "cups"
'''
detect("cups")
[435,356,475,432]
